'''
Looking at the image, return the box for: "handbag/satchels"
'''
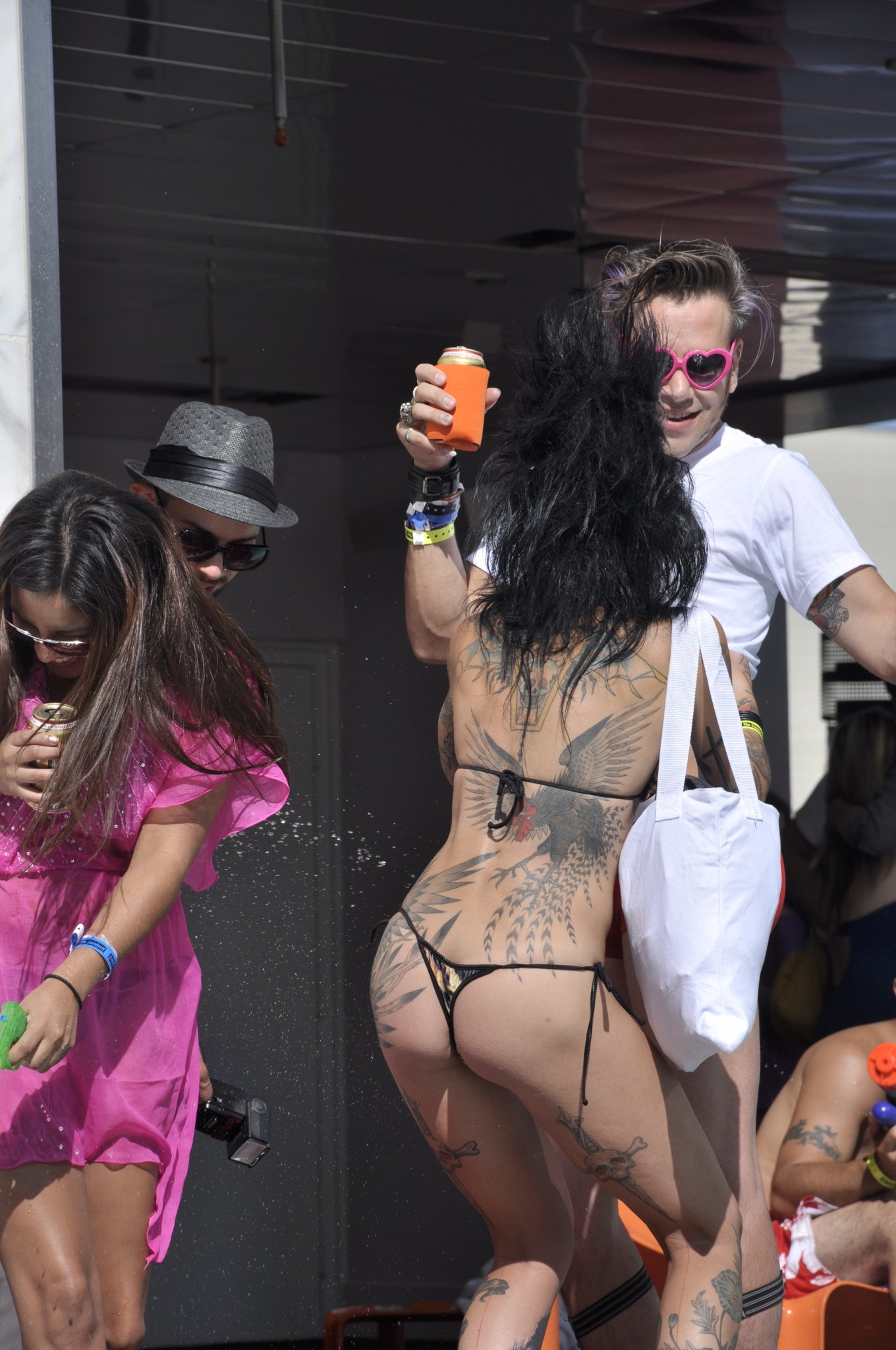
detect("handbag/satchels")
[616,606,784,1073]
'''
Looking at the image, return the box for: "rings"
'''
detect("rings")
[404,427,416,444]
[417,379,421,386]
[399,402,419,423]
[413,386,423,403]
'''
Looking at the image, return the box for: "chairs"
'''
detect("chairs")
[617,1200,896,1349]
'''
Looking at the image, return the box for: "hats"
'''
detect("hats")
[124,401,298,528]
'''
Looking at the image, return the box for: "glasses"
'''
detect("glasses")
[1,607,91,656]
[159,500,270,571]
[617,328,737,390]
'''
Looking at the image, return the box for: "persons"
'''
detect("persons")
[398,237,896,1350]
[122,401,297,1107]
[0,471,289,1350]
[752,664,895,1350]
[372,308,771,1350]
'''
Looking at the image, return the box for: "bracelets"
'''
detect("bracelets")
[43,974,83,1010]
[402,457,464,545]
[738,710,764,732]
[67,924,117,982]
[740,720,763,740]
[863,1151,895,1189]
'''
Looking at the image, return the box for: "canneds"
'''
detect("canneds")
[437,345,486,369]
[26,702,77,813]
